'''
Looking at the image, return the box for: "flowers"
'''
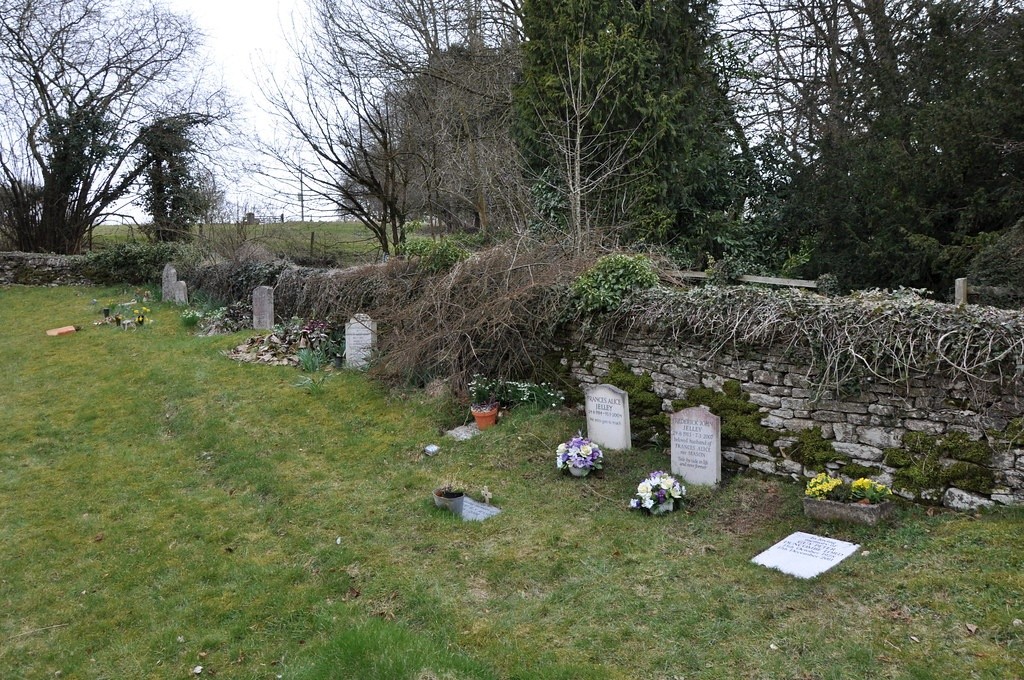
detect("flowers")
[555,430,606,477]
[179,309,202,326]
[133,307,150,323]
[628,470,688,517]
[469,373,496,409]
[805,472,893,504]
[497,378,566,411]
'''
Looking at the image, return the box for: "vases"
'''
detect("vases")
[137,316,145,325]
[568,466,592,477]
[470,406,498,431]
[801,496,895,528]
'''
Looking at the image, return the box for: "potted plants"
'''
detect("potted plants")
[431,468,469,520]
